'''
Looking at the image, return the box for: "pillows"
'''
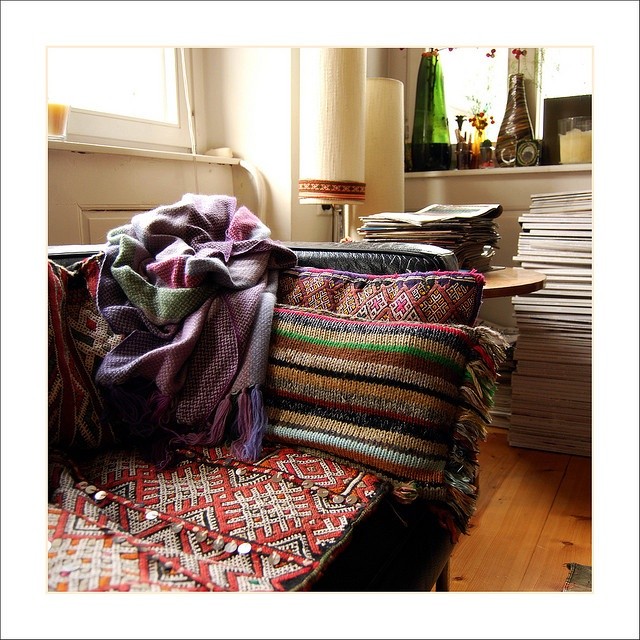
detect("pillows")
[260,297,507,543]
[49,251,124,464]
[280,265,485,330]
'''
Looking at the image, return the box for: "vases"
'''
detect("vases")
[495,74,535,167]
[409,53,453,171]
[471,130,485,169]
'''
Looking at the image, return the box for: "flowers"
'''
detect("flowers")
[398,48,455,142]
[453,115,467,137]
[469,111,495,136]
[485,48,528,74]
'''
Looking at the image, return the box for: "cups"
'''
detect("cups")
[48,97,73,142]
[557,116,592,165]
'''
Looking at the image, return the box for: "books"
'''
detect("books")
[473,317,520,430]
[356,203,507,273]
[507,190,592,459]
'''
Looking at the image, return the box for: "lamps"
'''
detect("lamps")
[341,75,406,242]
[297,47,368,241]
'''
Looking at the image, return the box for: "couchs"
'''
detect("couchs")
[49,231,479,591]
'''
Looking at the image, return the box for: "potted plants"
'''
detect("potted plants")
[479,139,495,163]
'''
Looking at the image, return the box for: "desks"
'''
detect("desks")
[475,265,550,300]
